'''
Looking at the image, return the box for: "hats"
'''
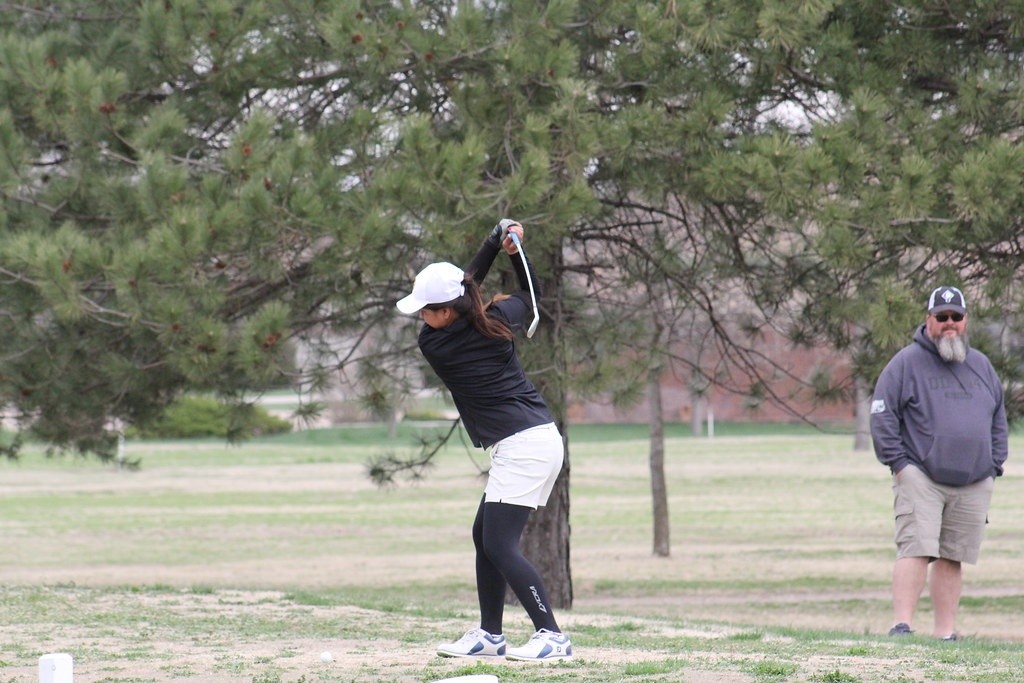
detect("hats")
[927,286,967,316]
[395,261,466,314]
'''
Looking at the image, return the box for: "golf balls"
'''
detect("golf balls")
[320,650,332,663]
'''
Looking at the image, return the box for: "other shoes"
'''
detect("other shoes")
[889,623,960,641]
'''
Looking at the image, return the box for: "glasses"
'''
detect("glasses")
[929,312,965,322]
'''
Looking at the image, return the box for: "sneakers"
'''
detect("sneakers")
[436,629,507,659]
[506,628,574,661]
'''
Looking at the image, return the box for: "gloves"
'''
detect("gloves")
[488,218,520,246]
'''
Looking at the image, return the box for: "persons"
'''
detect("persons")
[870,286,1008,641]
[395,218,573,662]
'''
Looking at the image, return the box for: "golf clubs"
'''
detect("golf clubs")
[509,231,540,339]
